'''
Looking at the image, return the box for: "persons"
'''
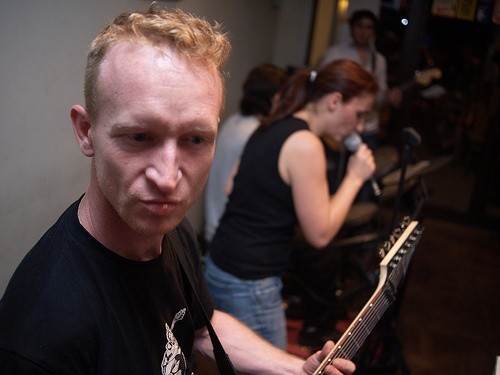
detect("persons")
[1,0,356,375]
[196,11,402,353]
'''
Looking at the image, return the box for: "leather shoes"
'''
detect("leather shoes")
[285,292,315,319]
[303,306,336,336]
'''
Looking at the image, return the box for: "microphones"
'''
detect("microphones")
[345,134,382,197]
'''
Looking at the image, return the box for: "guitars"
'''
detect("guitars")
[375,65,442,107]
[311,214,427,375]
